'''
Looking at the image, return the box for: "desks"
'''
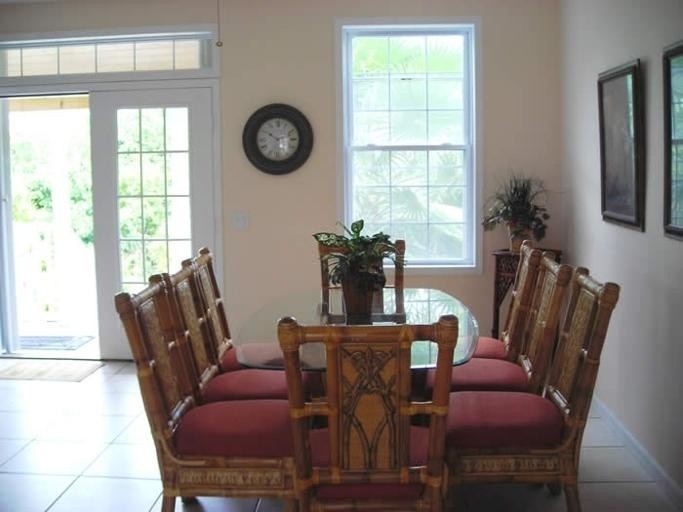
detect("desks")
[234,285,481,372]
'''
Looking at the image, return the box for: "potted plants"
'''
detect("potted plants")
[312,220,408,315]
[480,174,551,250]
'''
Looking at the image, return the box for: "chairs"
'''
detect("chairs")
[162,259,308,400]
[114,274,298,512]
[422,248,574,394]
[473,239,543,360]
[445,266,622,512]
[181,247,292,371]
[276,313,459,510]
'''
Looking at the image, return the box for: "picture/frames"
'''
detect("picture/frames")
[596,38,682,241]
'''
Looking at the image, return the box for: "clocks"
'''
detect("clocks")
[241,102,314,175]
